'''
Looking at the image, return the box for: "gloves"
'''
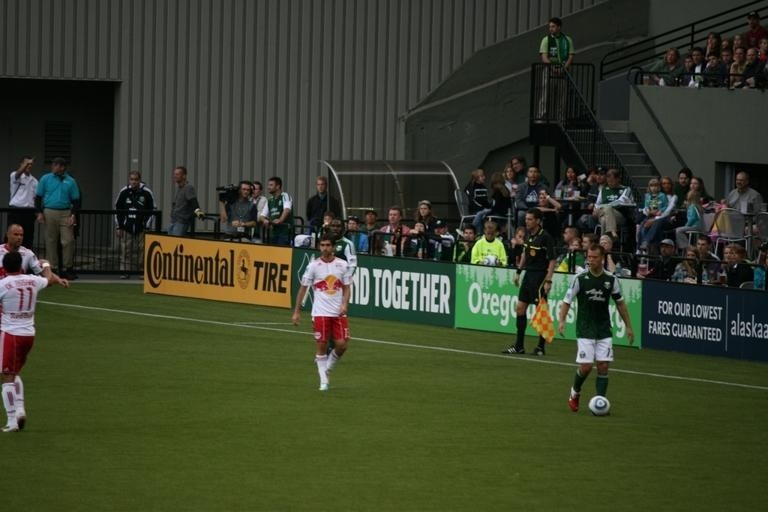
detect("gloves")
[194,207,206,218]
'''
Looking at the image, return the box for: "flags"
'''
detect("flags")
[530,299,559,342]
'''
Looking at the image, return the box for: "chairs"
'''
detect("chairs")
[446,182,767,295]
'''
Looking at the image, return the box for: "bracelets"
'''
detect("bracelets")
[41,263,51,267]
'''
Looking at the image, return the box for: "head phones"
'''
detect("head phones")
[238,181,255,197]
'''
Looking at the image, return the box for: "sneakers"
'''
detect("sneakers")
[15,410,26,429]
[327,346,333,355]
[53,270,144,280]
[502,344,525,354]
[0,423,19,432]
[530,345,545,356]
[318,381,332,391]
[325,351,338,376]
[568,385,580,411]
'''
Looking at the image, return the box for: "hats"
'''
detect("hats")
[433,217,447,227]
[51,157,66,164]
[660,238,675,246]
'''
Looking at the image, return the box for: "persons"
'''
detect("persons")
[115,171,157,278]
[0,224,69,288]
[292,234,351,391]
[649,10,768,88]
[559,245,634,412]
[168,166,205,236]
[8,156,38,249]
[502,207,557,356]
[537,17,575,126]
[0,252,54,432]
[34,155,81,280]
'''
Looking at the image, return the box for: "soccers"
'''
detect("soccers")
[589,395,610,416]
